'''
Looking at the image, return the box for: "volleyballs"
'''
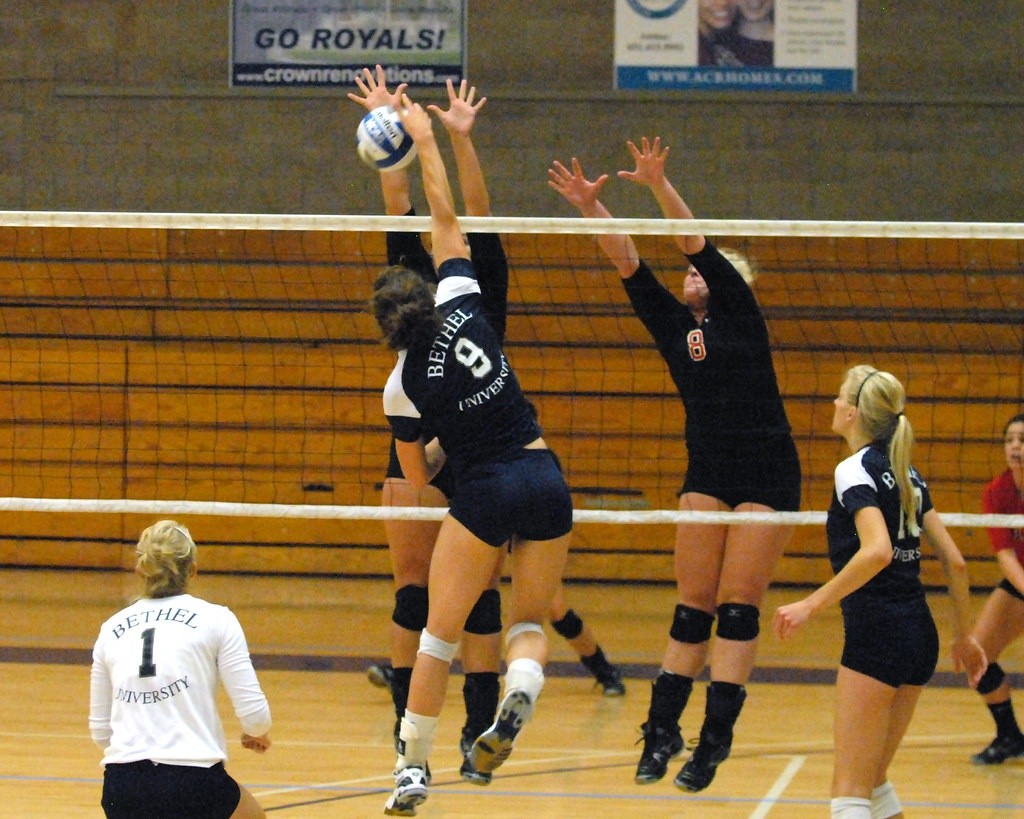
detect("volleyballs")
[353,105,419,175]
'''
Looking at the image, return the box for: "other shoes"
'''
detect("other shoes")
[366,658,391,686]
[597,670,625,696]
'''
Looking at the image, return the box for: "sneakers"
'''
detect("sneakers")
[384,763,429,816]
[634,722,685,785]
[971,734,1024,767]
[674,732,733,793]
[393,729,431,782]
[470,688,534,773]
[460,725,493,786]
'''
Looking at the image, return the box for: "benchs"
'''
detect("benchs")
[0,226,1020,591]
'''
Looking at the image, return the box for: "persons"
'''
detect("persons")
[347,63,627,817]
[968,413,1024,763]
[544,134,802,796]
[87,517,275,819]
[769,362,988,817]
